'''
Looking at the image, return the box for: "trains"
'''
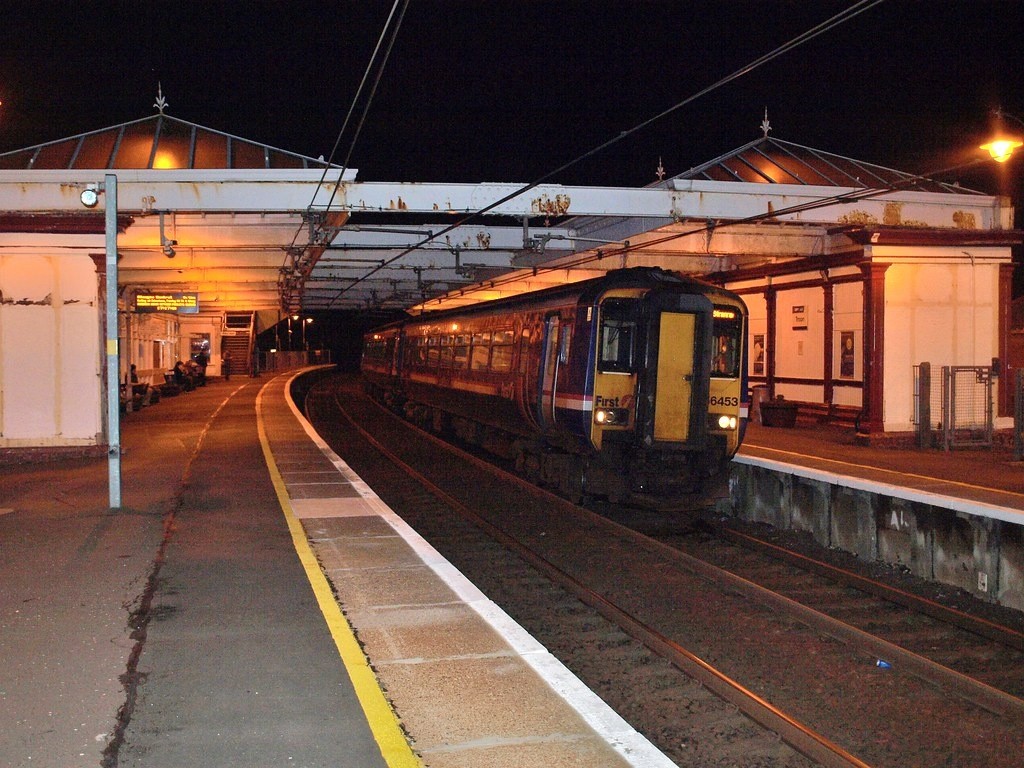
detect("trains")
[357,262,753,528]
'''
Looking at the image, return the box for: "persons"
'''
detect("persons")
[124,364,151,407]
[171,359,205,392]
[195,348,207,384]
[223,350,230,380]
